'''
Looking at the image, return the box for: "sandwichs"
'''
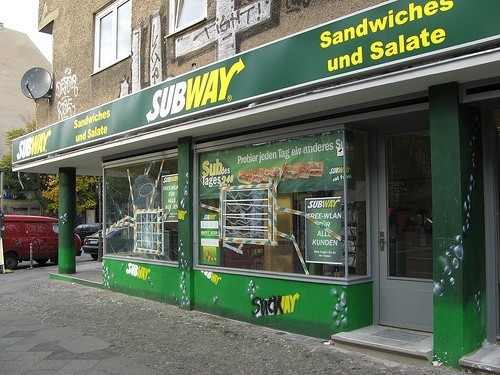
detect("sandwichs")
[237,162,324,185]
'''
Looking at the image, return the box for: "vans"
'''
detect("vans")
[0,213,81,269]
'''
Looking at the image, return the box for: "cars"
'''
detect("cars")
[74,225,100,245]
[83,225,134,260]
